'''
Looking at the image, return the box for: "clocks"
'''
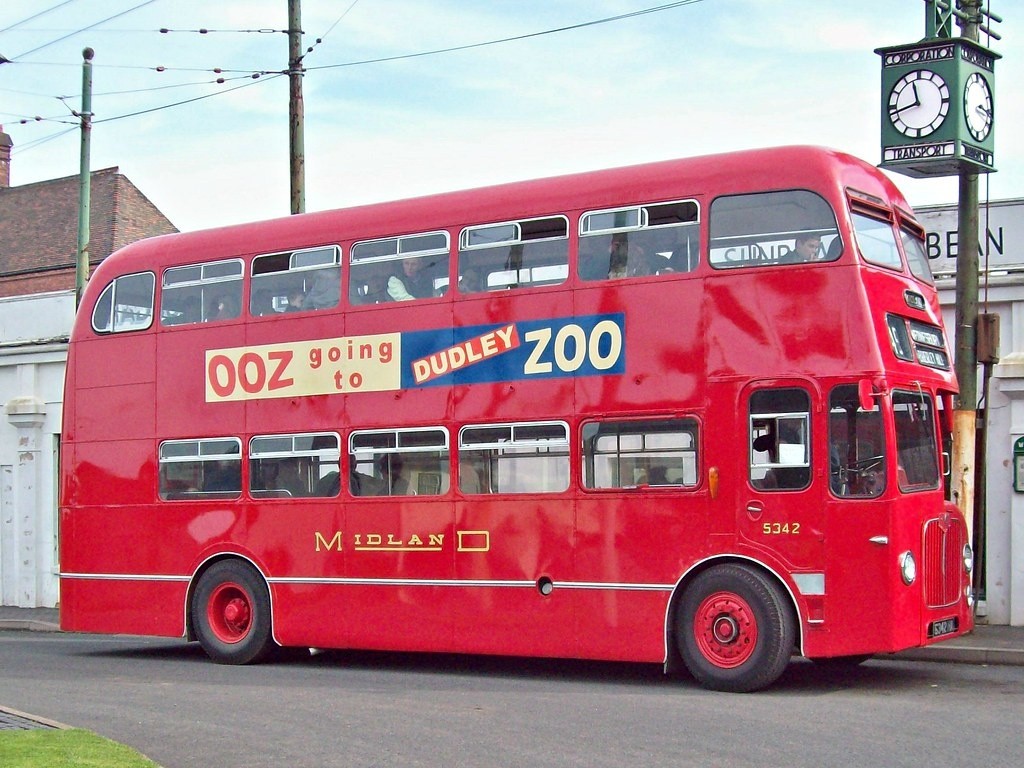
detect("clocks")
[963,72,994,142]
[887,69,951,139]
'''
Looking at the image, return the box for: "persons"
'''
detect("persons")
[161,228,843,327]
[168,423,848,498]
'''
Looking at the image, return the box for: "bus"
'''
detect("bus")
[54,144,975,693]
